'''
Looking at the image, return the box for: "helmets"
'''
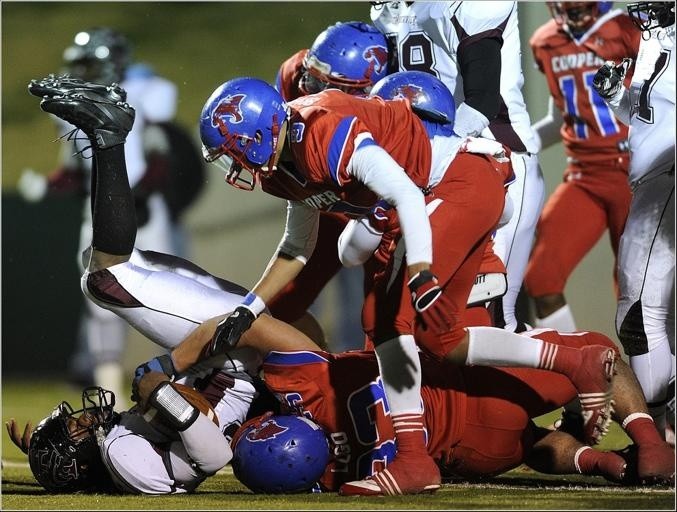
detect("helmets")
[649,0,675,28]
[29,401,101,492]
[200,77,291,166]
[63,28,130,85]
[546,0,599,15]
[597,1,612,14]
[302,22,390,88]
[231,415,329,494]
[369,70,455,124]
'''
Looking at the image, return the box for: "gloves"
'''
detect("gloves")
[131,353,178,408]
[205,292,265,357]
[406,271,456,334]
[592,58,632,99]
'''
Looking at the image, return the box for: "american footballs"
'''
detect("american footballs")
[138,379,219,438]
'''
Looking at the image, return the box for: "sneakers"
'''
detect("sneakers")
[28,72,135,149]
[338,454,441,496]
[572,345,615,444]
[598,444,675,485]
